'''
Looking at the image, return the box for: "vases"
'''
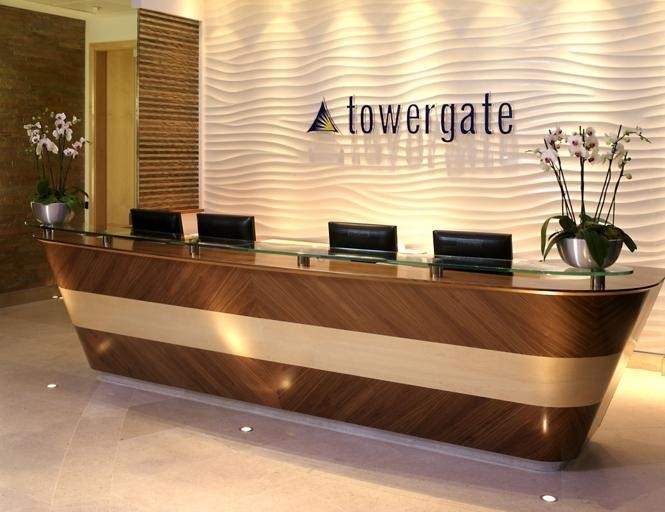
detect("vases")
[555,237,622,271]
[31,202,68,227]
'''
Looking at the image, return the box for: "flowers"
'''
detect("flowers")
[22,106,93,215]
[523,124,653,267]
